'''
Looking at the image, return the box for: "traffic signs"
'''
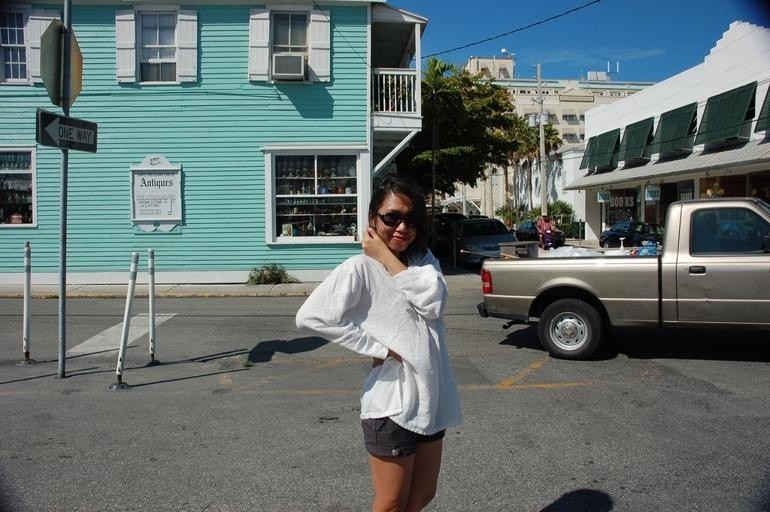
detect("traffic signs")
[34,107,98,151]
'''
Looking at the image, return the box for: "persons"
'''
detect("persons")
[536,213,552,247]
[293,174,464,512]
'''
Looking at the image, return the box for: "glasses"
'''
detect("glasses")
[377,209,421,227]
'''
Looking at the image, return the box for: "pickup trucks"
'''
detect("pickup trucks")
[479,199,770,358]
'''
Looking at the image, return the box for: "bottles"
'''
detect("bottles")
[0,154,31,204]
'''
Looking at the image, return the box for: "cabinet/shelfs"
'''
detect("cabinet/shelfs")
[275,154,357,235]
[2,153,32,224]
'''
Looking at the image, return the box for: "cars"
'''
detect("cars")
[598,219,664,248]
[425,204,567,269]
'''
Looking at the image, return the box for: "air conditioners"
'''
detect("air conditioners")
[271,53,307,81]
